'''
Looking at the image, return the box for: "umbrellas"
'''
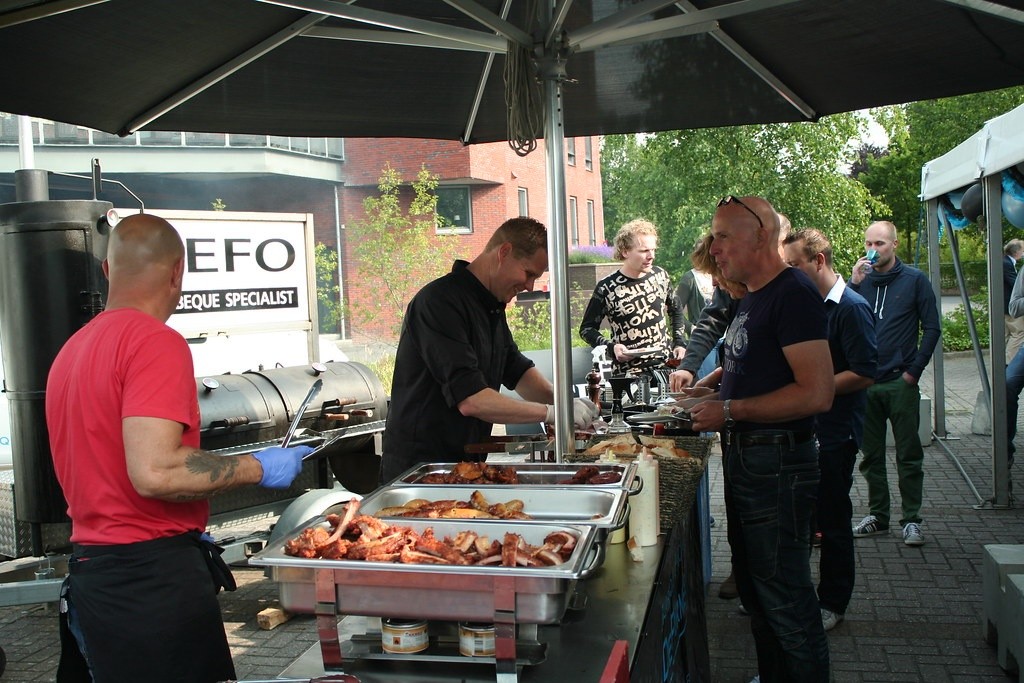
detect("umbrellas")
[0,0,1024,463]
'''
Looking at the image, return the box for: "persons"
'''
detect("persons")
[45,214,316,683]
[379,216,600,487]
[579,218,688,377]
[1001,238,1024,492]
[671,195,836,683]
[848,222,940,545]
[668,210,880,632]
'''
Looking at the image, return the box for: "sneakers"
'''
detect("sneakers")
[852,515,889,537]
[903,522,925,544]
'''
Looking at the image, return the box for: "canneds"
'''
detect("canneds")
[381,617,428,653]
[456,621,495,657]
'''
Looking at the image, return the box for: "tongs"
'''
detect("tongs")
[282,379,348,460]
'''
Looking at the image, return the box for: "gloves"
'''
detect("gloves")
[251,445,315,490]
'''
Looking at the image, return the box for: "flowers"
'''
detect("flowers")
[568,239,617,264]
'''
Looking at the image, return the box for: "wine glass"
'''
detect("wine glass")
[860,249,880,274]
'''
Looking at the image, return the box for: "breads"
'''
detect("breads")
[584,432,689,457]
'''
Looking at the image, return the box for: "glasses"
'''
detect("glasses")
[716,194,764,230]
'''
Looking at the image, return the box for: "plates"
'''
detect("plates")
[627,412,680,421]
[668,393,686,396]
[622,347,663,356]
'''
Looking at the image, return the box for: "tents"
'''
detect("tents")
[921,102,1024,510]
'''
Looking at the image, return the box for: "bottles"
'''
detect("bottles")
[594,446,660,546]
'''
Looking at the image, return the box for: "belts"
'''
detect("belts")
[719,425,814,446]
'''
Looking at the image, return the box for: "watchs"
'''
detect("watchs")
[724,399,735,429]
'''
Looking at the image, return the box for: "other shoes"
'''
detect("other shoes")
[720,572,739,599]
[710,516,715,528]
[820,609,845,630]
